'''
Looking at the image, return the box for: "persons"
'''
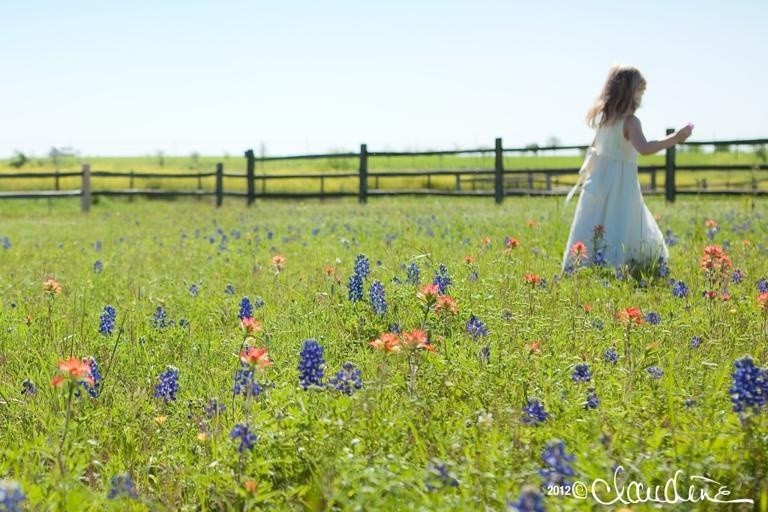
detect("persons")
[560,63,695,273]
